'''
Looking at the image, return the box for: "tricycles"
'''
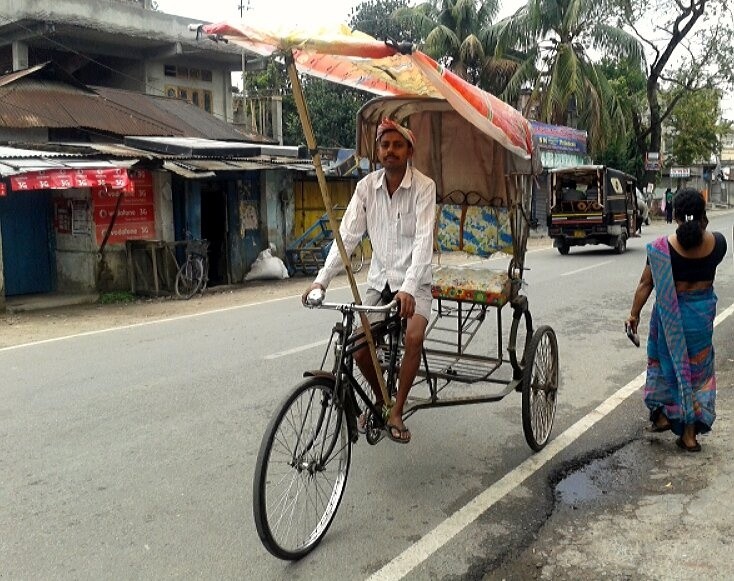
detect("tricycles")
[252,93,560,561]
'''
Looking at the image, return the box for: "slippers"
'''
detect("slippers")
[676,436,701,452]
[357,415,368,433]
[387,425,411,443]
[646,421,671,432]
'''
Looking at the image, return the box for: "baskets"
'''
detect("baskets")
[187,240,209,257]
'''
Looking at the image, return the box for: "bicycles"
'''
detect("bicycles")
[174,231,213,301]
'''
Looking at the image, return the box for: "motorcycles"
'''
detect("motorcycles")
[546,165,638,256]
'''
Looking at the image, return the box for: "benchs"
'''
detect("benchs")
[431,190,527,307]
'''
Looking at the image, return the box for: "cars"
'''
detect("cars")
[635,187,651,226]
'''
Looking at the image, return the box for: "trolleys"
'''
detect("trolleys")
[284,202,365,278]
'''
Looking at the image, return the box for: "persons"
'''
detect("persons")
[302,118,437,444]
[562,182,598,215]
[625,187,728,452]
